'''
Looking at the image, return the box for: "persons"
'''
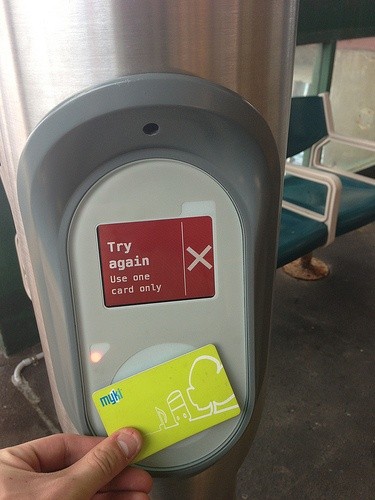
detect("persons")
[0,404,156,500]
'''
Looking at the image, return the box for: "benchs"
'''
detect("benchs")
[277,90,375,282]
